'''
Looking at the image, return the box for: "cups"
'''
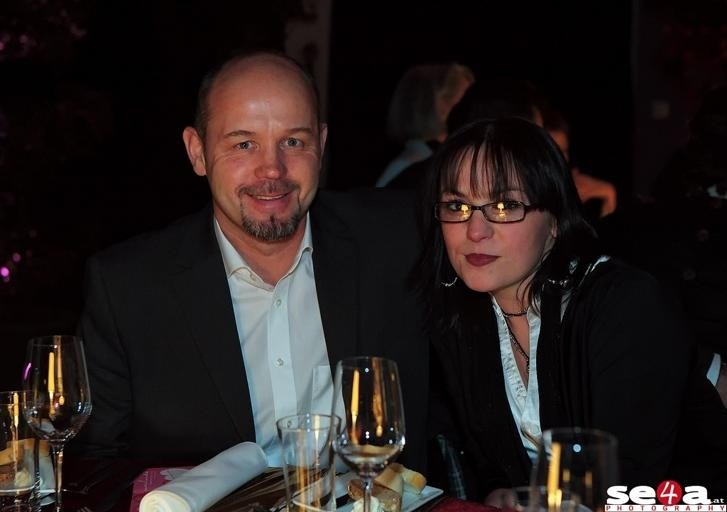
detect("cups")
[0,391,38,512]
[533,432,619,512]
[274,412,342,512]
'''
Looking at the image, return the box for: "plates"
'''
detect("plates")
[288,458,443,512]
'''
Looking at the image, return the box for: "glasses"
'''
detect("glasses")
[434,200,539,223]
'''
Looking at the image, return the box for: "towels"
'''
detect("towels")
[138,441,269,512]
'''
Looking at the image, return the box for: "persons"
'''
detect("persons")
[3,17,725,510]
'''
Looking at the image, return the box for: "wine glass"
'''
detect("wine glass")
[329,356,411,512]
[23,331,91,512]
